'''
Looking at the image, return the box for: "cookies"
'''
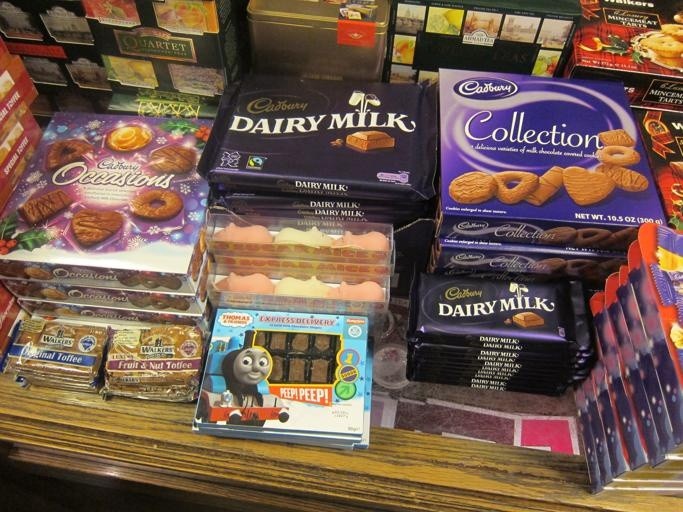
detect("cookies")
[11,319,202,393]
[16,125,195,248]
[448,128,649,205]
